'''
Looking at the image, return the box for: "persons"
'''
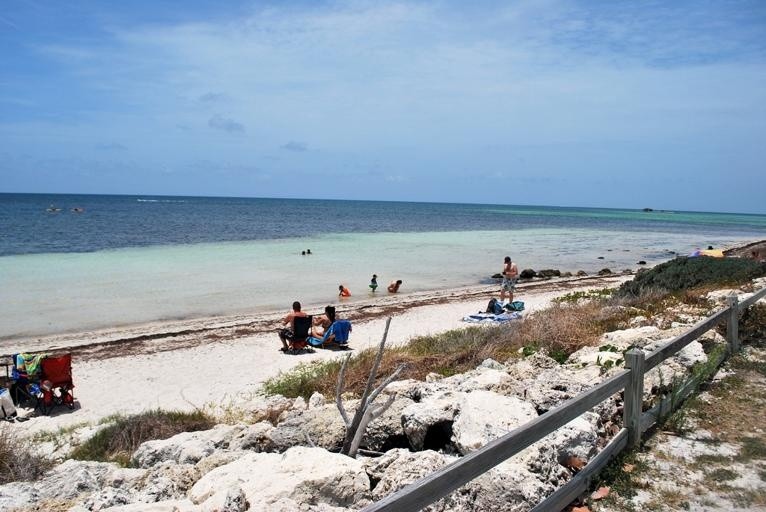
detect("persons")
[388,280,402,293]
[312,306,341,339]
[338,285,352,296]
[500,256,519,305]
[279,302,307,351]
[46,207,84,213]
[369,274,378,292]
[301,249,311,255]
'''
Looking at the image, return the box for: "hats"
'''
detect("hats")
[504,256,511,264]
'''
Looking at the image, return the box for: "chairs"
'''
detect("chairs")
[283,315,313,354]
[305,320,351,350]
[16,354,75,416]
